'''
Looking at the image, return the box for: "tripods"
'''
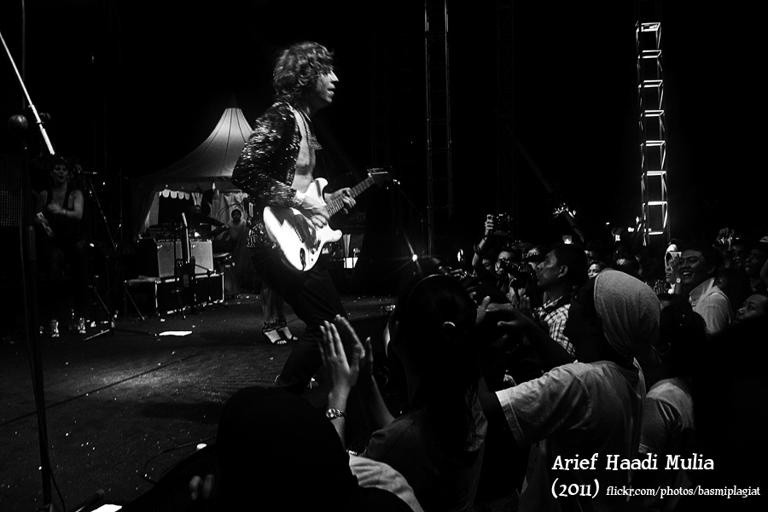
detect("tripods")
[86,176,112,317]
[114,208,146,323]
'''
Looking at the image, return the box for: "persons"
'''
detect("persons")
[228,41,360,388]
[23,153,88,343]
[70,196,767,508]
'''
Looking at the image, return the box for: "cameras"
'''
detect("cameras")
[508,262,536,290]
[493,209,513,233]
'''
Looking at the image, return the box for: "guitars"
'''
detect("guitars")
[263,167,389,272]
[176,213,197,297]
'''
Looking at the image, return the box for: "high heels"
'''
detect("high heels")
[261,323,299,347]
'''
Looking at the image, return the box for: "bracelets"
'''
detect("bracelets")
[63,208,67,215]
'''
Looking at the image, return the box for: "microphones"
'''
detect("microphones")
[76,170,98,176]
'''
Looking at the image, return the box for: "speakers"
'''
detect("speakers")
[189,238,214,275]
[134,239,183,278]
